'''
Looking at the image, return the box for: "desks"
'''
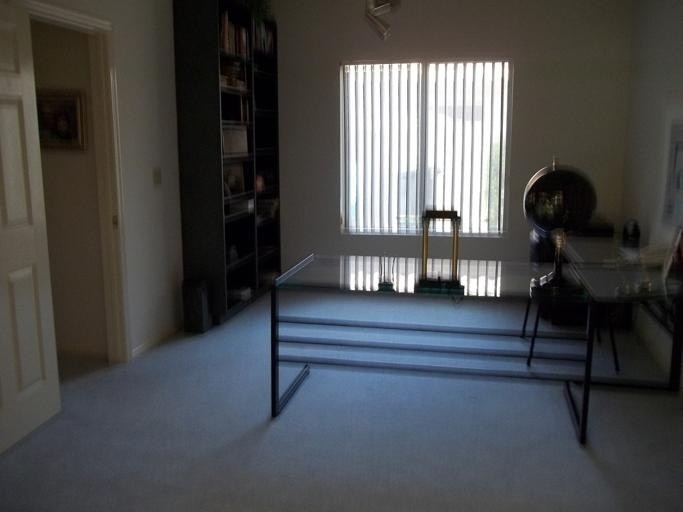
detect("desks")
[554,236,682,446]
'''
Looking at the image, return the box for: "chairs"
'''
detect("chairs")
[513,219,623,374]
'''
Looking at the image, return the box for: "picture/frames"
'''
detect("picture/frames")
[37,88,88,153]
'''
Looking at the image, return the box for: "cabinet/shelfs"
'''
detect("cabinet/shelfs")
[171,0,282,335]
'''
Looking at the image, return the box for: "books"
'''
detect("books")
[221,6,273,123]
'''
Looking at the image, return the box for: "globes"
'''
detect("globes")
[523,160,597,301]
